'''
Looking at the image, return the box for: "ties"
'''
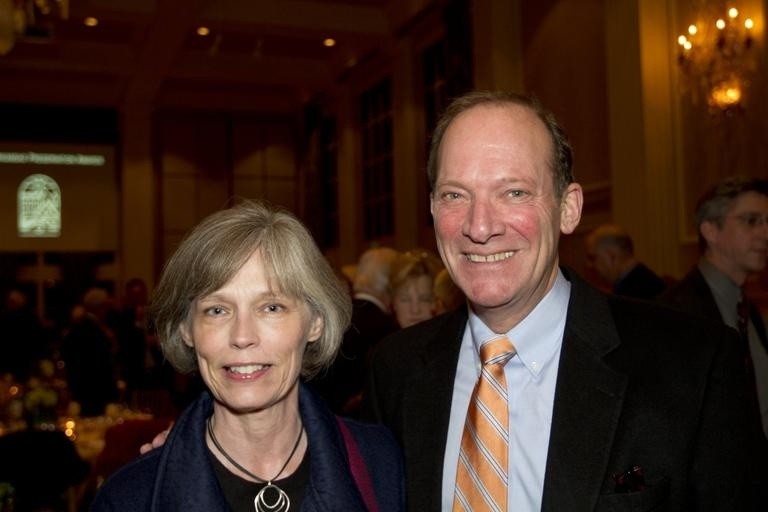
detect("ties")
[735,295,755,348]
[450,334,518,511]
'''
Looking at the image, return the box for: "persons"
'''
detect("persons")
[299,247,466,424]
[584,224,666,301]
[1,277,206,512]
[139,89,768,512]
[654,176,768,440]
[92,196,409,512]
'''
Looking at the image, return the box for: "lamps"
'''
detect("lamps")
[677,8,756,115]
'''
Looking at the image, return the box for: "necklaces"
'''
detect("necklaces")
[208,411,304,512]
